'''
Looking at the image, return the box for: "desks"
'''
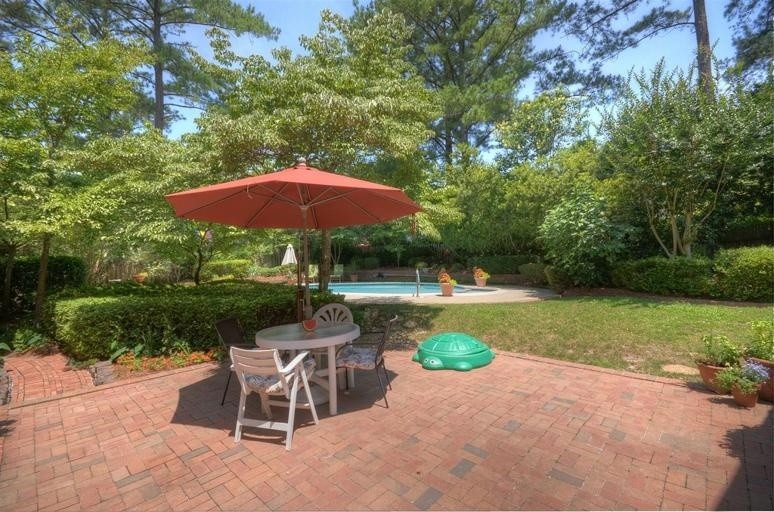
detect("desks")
[254,323,360,416]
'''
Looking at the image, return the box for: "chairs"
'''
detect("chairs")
[224,341,319,451]
[329,263,344,283]
[335,314,399,408]
[213,317,261,412]
[308,264,319,283]
[310,302,353,392]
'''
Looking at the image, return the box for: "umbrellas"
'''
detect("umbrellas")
[163,156,428,323]
[280,243,298,273]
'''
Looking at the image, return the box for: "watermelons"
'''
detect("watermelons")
[301,319,319,331]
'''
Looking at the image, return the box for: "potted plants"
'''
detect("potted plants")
[287,271,295,284]
[437,271,457,297]
[349,260,360,281]
[473,269,490,287]
[696,318,774,407]
